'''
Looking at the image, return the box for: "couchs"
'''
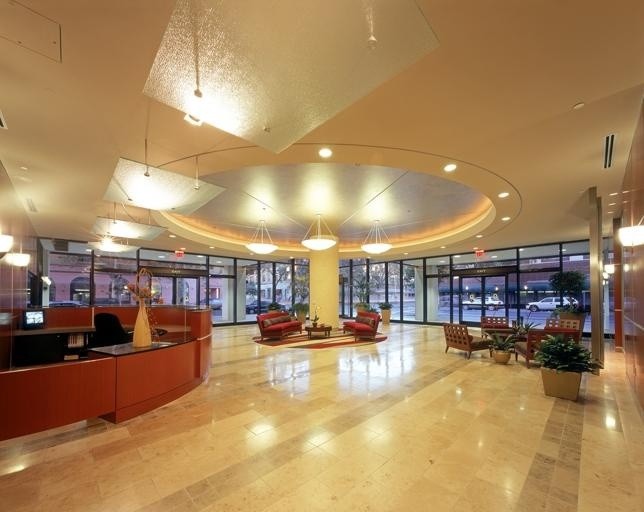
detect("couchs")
[256,312,302,340]
[343,312,381,343]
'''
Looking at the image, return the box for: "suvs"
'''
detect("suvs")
[525,296,579,312]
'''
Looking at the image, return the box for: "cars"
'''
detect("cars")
[48,300,91,306]
[439,295,459,307]
[461,296,505,311]
[244,299,289,314]
[200,298,221,310]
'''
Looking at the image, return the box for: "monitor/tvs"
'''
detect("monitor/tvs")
[23,310,45,329]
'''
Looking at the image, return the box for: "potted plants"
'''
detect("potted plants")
[347,275,377,311]
[480,331,519,365]
[287,273,309,323]
[378,302,393,332]
[548,269,591,340]
[267,301,281,314]
[534,333,604,402]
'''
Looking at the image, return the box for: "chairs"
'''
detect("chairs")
[481,317,509,338]
[546,318,581,330]
[94,313,133,345]
[443,323,494,359]
[515,329,580,368]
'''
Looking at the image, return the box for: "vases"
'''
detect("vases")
[312,323,317,327]
[133,297,152,347]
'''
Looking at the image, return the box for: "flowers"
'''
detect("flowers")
[310,306,321,322]
[127,282,162,300]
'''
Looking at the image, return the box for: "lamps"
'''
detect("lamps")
[360,220,393,254]
[245,219,278,254]
[301,213,336,251]
[0,234,14,253]
[89,236,140,253]
[4,237,30,267]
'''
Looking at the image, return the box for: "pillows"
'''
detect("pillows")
[263,315,291,327]
[355,316,375,328]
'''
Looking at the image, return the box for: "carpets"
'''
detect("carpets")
[251,327,389,349]
